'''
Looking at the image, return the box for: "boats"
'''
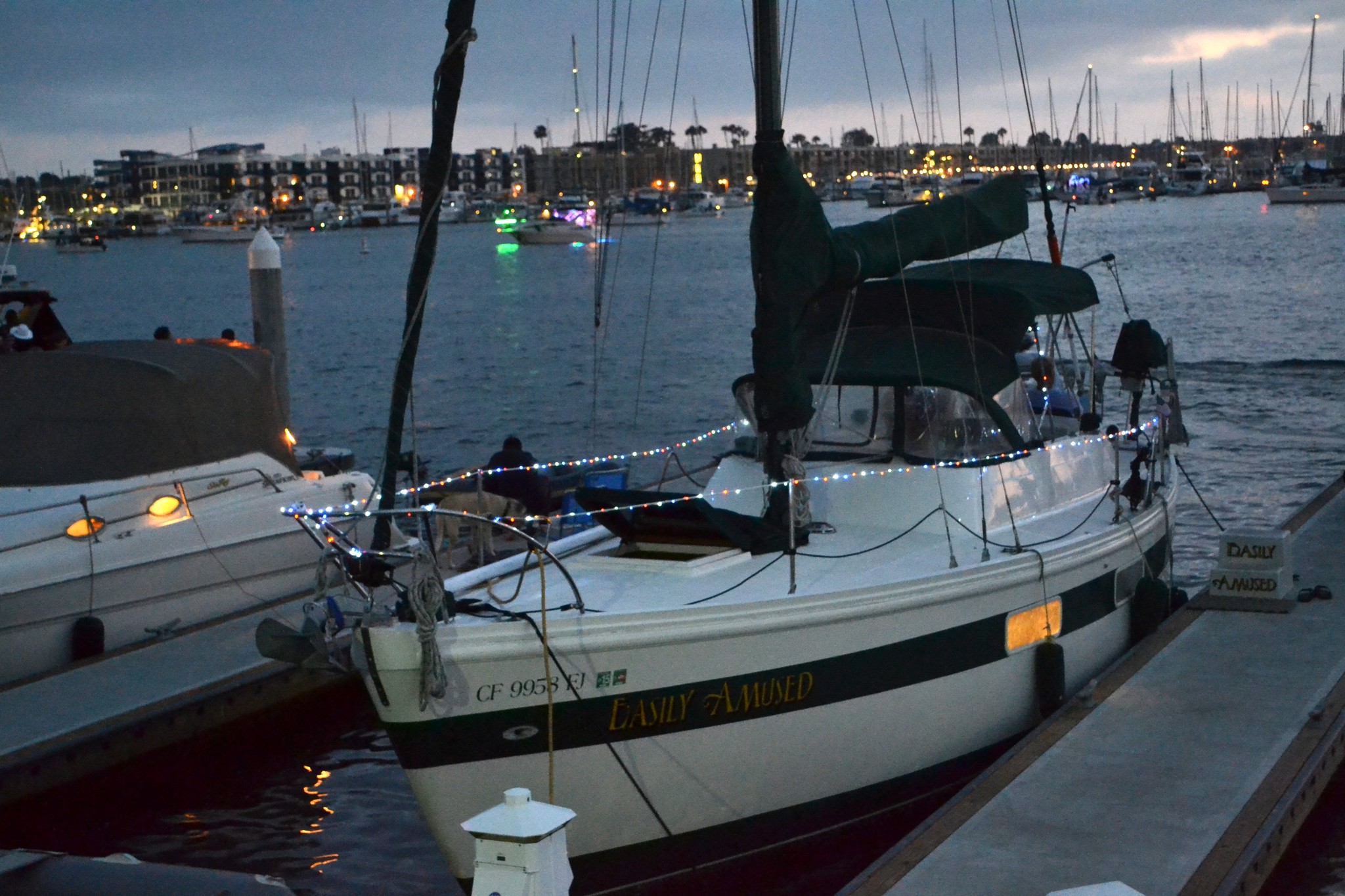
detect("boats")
[1,0,1344,895]
